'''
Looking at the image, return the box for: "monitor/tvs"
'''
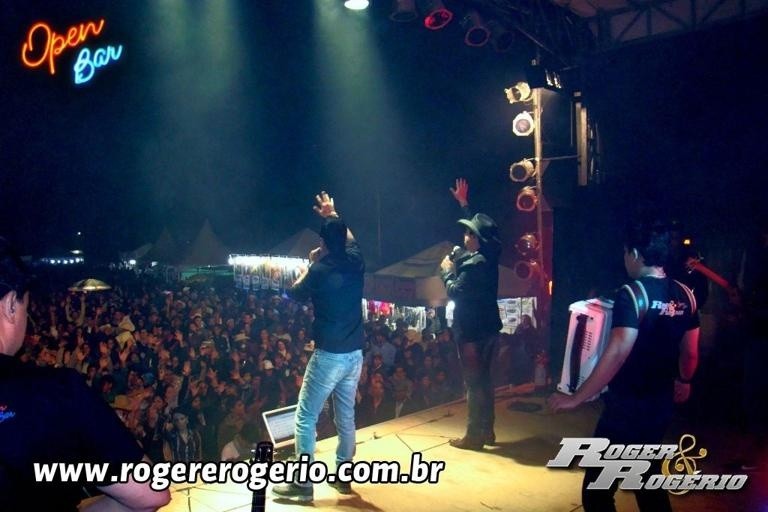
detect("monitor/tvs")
[262,404,319,449]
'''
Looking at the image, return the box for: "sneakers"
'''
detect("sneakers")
[328,479,350,494]
[480,434,495,444]
[273,483,313,501]
[449,437,481,449]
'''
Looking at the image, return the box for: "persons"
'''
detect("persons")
[547,218,702,511]
[2,258,539,497]
[269,189,365,500]
[439,177,502,453]
[4,260,172,511]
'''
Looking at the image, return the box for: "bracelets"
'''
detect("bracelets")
[677,375,690,385]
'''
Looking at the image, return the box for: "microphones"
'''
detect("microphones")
[450,246,463,261]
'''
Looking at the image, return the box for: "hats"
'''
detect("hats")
[457,212,500,247]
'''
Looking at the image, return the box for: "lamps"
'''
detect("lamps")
[389,2,491,49]
[503,81,544,282]
